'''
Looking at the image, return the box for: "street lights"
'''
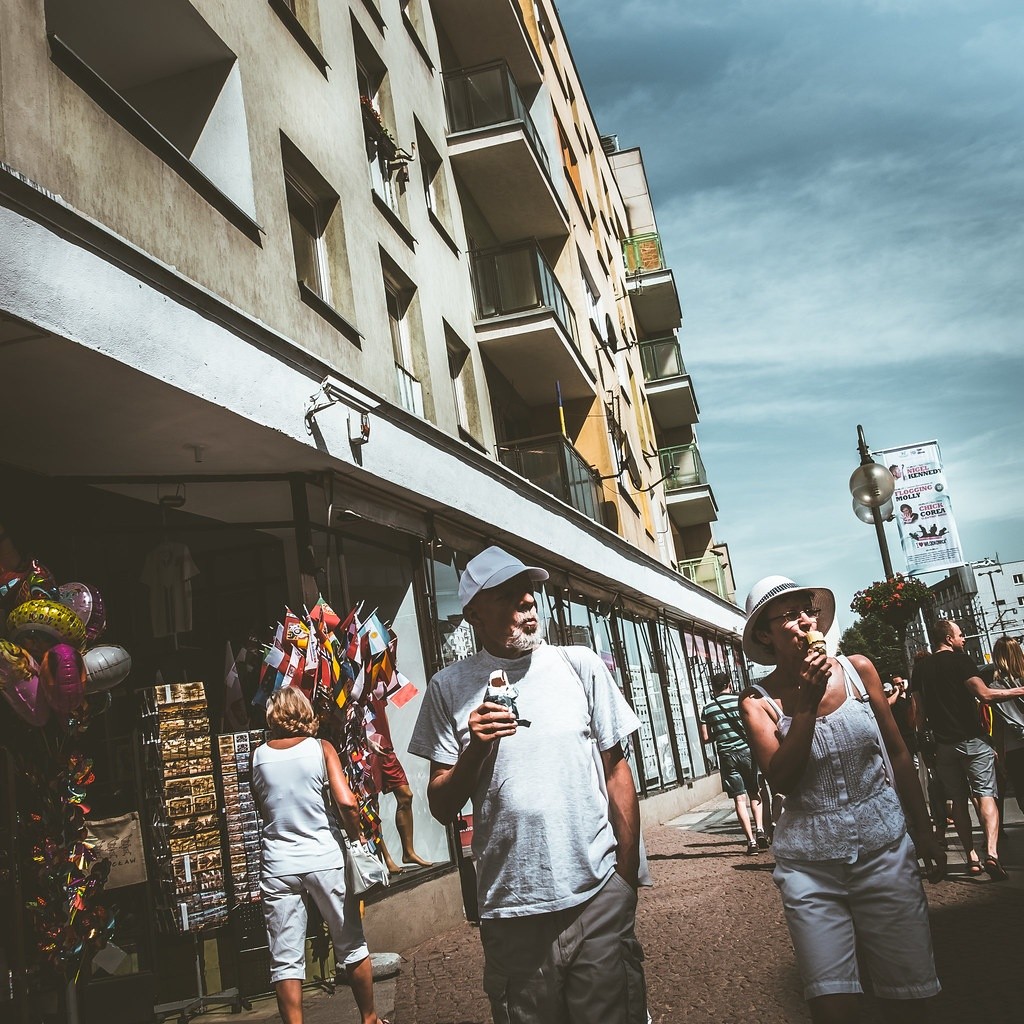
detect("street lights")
[847,423,913,695]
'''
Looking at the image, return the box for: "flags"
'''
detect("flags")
[254,594,418,716]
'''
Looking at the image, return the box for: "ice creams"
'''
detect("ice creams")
[806,631,826,656]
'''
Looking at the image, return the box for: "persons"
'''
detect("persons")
[918,621,1024,879]
[989,635,1024,837]
[699,673,769,855]
[737,575,948,1024]
[408,546,654,1024]
[365,698,432,874]
[251,685,391,1023]
[757,768,783,843]
[884,647,1010,838]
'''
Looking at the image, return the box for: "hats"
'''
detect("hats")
[742,575,836,666]
[711,672,730,689]
[458,546,549,612]
[883,683,894,692]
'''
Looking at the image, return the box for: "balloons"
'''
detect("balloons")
[0,558,137,732]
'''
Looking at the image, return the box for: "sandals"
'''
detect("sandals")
[966,857,986,877]
[984,855,1009,883]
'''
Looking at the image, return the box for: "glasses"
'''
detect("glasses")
[764,608,821,625]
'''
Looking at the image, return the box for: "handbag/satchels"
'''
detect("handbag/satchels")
[345,839,390,901]
[888,780,922,860]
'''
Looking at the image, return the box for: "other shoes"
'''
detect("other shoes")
[981,841,988,850]
[998,829,1007,839]
[937,839,948,852]
[746,842,758,855]
[756,838,769,849]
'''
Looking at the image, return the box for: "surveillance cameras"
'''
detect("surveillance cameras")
[321,374,382,414]
